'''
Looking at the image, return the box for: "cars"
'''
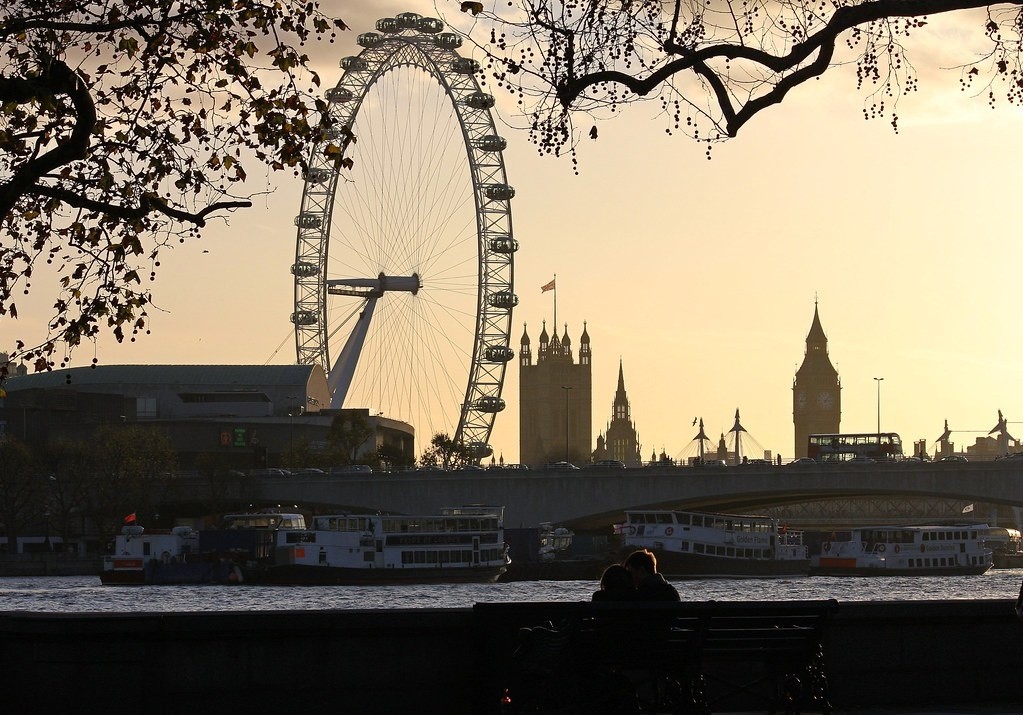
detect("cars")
[333,464,373,477]
[488,466,503,472]
[252,467,293,477]
[463,464,484,472]
[295,467,327,478]
[419,466,446,473]
[152,472,177,479]
[503,452,1023,473]
[213,470,247,479]
[25,473,57,482]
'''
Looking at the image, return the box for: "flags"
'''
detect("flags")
[540,279,555,293]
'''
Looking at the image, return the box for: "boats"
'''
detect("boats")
[814,522,995,577]
[97,507,308,587]
[985,527,1023,568]
[612,509,813,581]
[276,503,513,587]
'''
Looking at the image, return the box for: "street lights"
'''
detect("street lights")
[19,404,32,441]
[286,395,297,469]
[561,386,572,463]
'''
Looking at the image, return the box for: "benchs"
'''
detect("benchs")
[468,599,839,715]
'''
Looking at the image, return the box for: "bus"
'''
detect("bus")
[807,432,903,464]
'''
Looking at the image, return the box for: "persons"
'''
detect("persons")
[592,564,649,671]
[624,550,684,666]
